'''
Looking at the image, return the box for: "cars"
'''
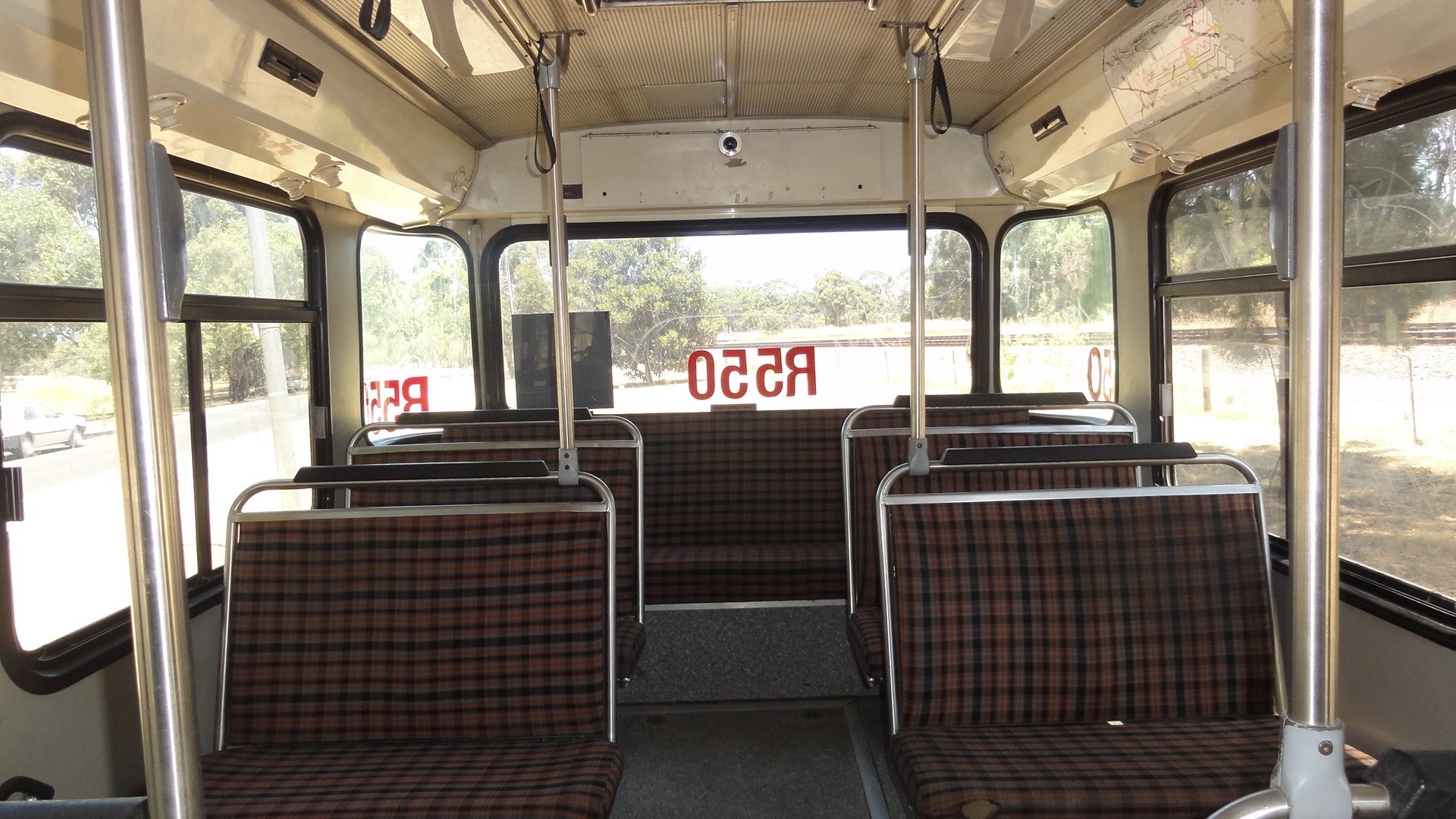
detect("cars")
[1,400,89,460]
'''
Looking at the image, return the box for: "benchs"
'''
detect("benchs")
[199,394,1384,819]
